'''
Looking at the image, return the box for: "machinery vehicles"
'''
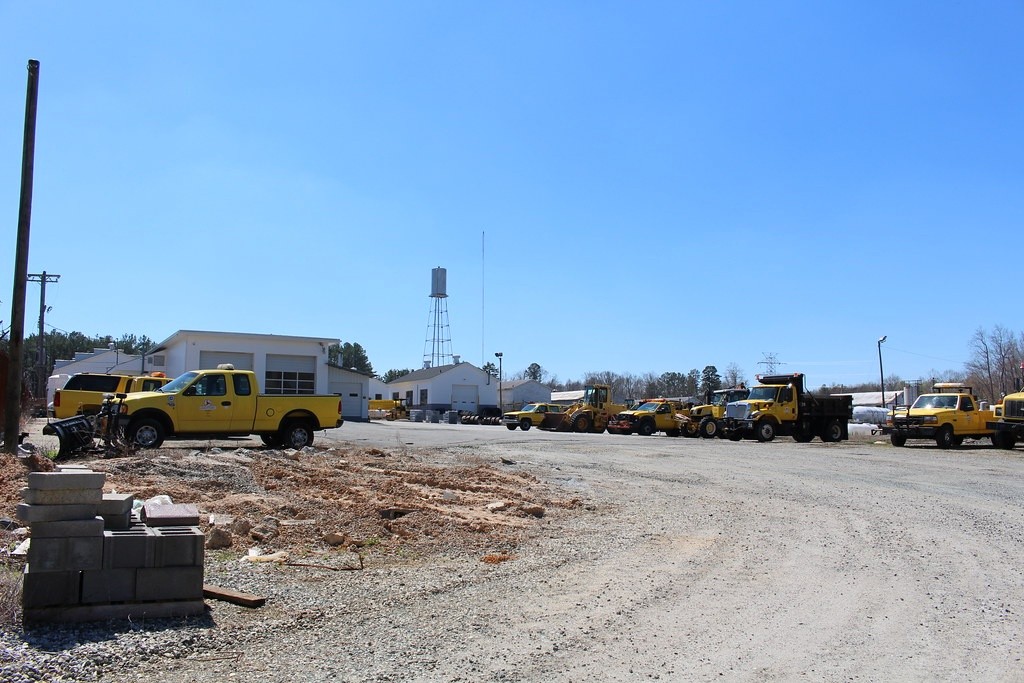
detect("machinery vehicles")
[386,398,410,421]
[681,389,751,438]
[537,384,628,433]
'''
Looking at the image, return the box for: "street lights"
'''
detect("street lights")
[140,343,149,372]
[878,336,887,408]
[495,353,503,416]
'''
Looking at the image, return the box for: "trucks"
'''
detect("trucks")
[726,373,853,442]
[986,362,1024,449]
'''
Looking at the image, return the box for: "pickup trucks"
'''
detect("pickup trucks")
[503,403,566,431]
[102,364,344,450]
[871,383,1001,447]
[48,372,174,419]
[608,400,692,437]
